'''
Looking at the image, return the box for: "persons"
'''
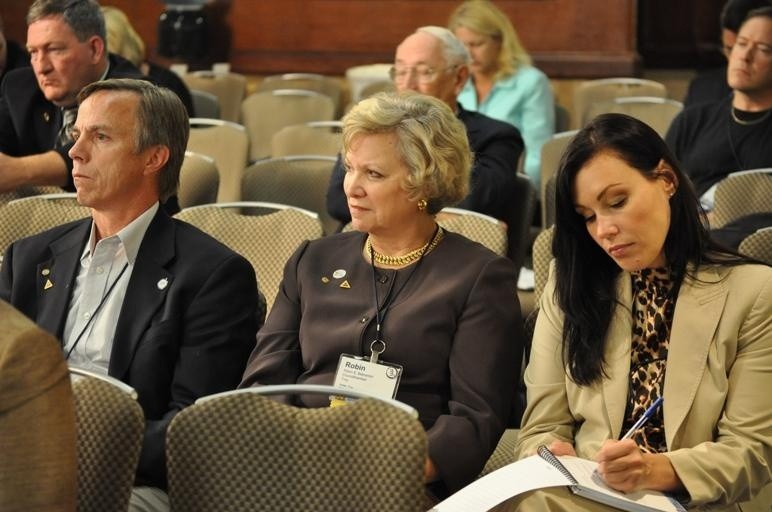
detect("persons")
[237,87,526,500]
[1,80,259,511]
[500,114,772,511]
[682,1,771,110]
[664,5,772,198]
[448,1,556,229]
[326,27,525,257]
[1,0,194,217]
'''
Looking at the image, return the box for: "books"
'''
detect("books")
[431,445,685,512]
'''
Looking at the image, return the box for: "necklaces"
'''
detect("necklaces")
[366,228,444,266]
[66,263,129,356]
[731,107,770,124]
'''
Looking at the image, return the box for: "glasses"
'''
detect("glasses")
[388,61,457,84]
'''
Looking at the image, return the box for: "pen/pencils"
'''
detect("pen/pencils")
[593,398,664,474]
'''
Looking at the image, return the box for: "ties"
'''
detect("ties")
[54,107,79,150]
